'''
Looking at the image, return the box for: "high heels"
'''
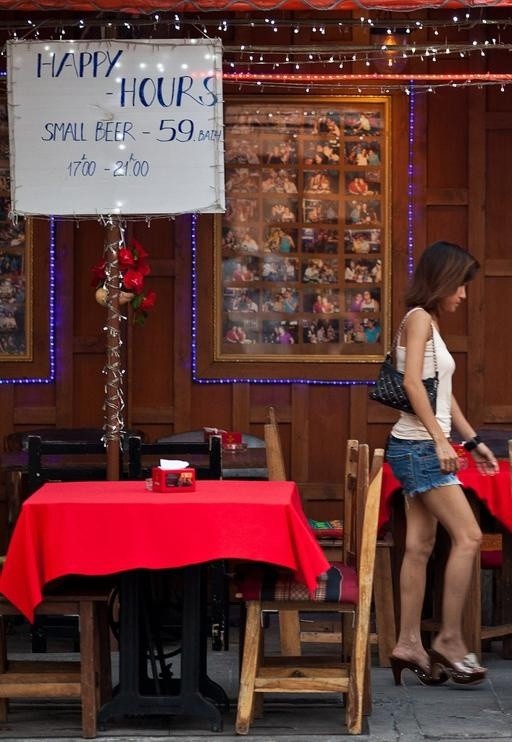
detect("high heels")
[389,656,450,687]
[428,647,487,686]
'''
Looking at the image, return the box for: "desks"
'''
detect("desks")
[378,456,512,663]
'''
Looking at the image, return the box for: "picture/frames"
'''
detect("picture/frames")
[0,77,51,379]
[197,79,409,380]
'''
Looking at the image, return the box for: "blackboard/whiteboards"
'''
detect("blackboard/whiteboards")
[7,38,226,216]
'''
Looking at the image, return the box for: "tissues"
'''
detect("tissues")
[151,457,197,493]
[203,425,243,447]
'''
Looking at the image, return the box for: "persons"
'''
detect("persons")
[223,109,381,343]
[385,241,500,686]
[0,106,26,355]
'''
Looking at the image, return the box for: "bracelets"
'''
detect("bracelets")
[463,435,484,452]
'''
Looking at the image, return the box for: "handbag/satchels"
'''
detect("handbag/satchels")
[368,307,438,415]
